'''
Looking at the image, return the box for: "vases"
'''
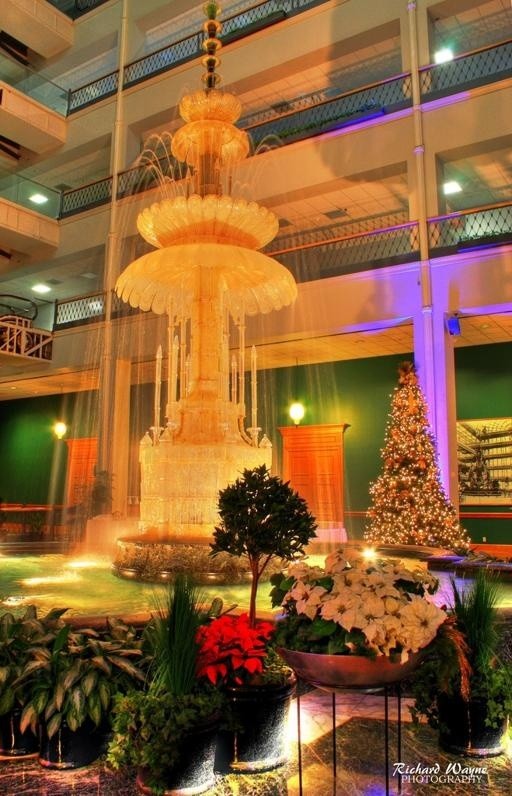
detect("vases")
[273,644,417,692]
[216,682,297,763]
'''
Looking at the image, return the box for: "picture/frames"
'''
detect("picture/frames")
[456,417,512,507]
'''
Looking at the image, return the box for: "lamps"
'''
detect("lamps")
[288,357,307,427]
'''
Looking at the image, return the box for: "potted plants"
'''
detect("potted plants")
[0,604,69,761]
[401,564,512,757]
[19,615,148,767]
[106,569,223,796]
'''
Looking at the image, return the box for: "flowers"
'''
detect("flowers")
[194,613,293,688]
[269,545,447,664]
